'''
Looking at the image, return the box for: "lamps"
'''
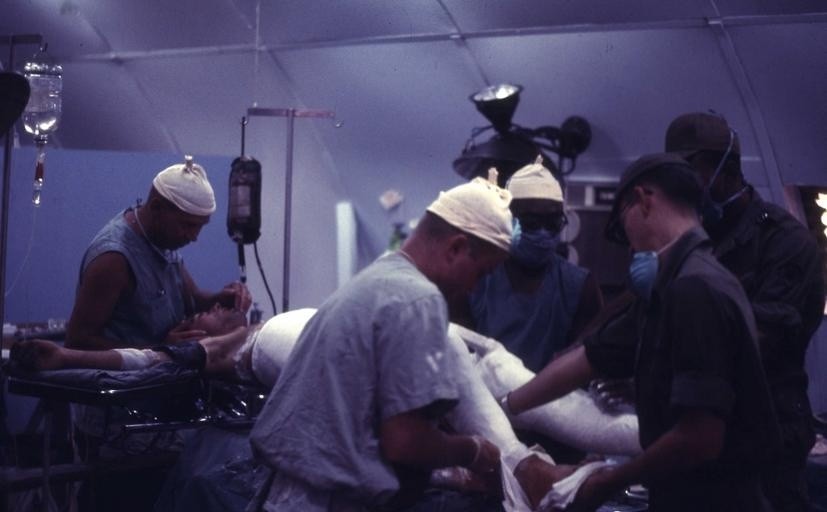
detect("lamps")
[469,79,591,165]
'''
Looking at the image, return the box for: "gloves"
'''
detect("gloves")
[466,433,502,475]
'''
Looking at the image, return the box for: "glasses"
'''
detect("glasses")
[520,212,569,235]
[606,187,653,244]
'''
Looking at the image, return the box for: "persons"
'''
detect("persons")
[11,303,646,511]
[63,159,250,510]
[245,176,514,511]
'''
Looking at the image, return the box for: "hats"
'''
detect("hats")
[423,166,516,255]
[151,155,218,218]
[602,149,700,247]
[664,110,743,161]
[502,154,565,203]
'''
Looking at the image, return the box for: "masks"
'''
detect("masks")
[625,237,676,302]
[512,226,559,269]
